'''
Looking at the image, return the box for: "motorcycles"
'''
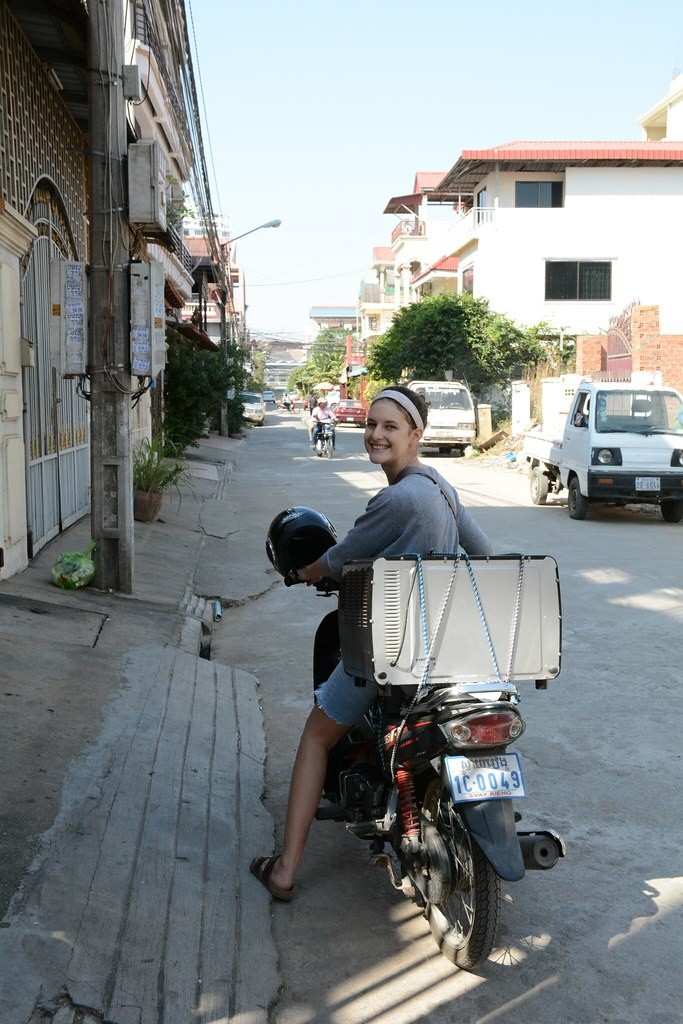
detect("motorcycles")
[283,552,567,971]
[309,415,339,459]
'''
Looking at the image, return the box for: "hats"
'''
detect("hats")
[318,397,328,404]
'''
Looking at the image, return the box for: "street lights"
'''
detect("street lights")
[220,218,281,438]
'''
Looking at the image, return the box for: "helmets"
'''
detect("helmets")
[266,506,340,585]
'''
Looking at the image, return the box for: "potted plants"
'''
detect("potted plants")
[134,424,204,521]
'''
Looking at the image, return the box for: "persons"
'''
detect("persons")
[251,388,493,898]
[283,393,339,451]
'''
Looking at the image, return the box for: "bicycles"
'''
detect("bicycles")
[276,400,301,414]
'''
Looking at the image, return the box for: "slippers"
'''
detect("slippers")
[250,853,295,900]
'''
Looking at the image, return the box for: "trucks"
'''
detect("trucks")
[521,380,683,522]
[406,380,477,456]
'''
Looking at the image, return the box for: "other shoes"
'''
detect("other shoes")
[311,443,315,450]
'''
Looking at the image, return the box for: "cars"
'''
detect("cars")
[237,391,267,426]
[303,395,312,410]
[335,398,366,428]
[288,391,300,401]
[262,391,276,404]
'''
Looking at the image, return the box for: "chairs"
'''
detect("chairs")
[587,398,606,418]
[631,400,653,420]
